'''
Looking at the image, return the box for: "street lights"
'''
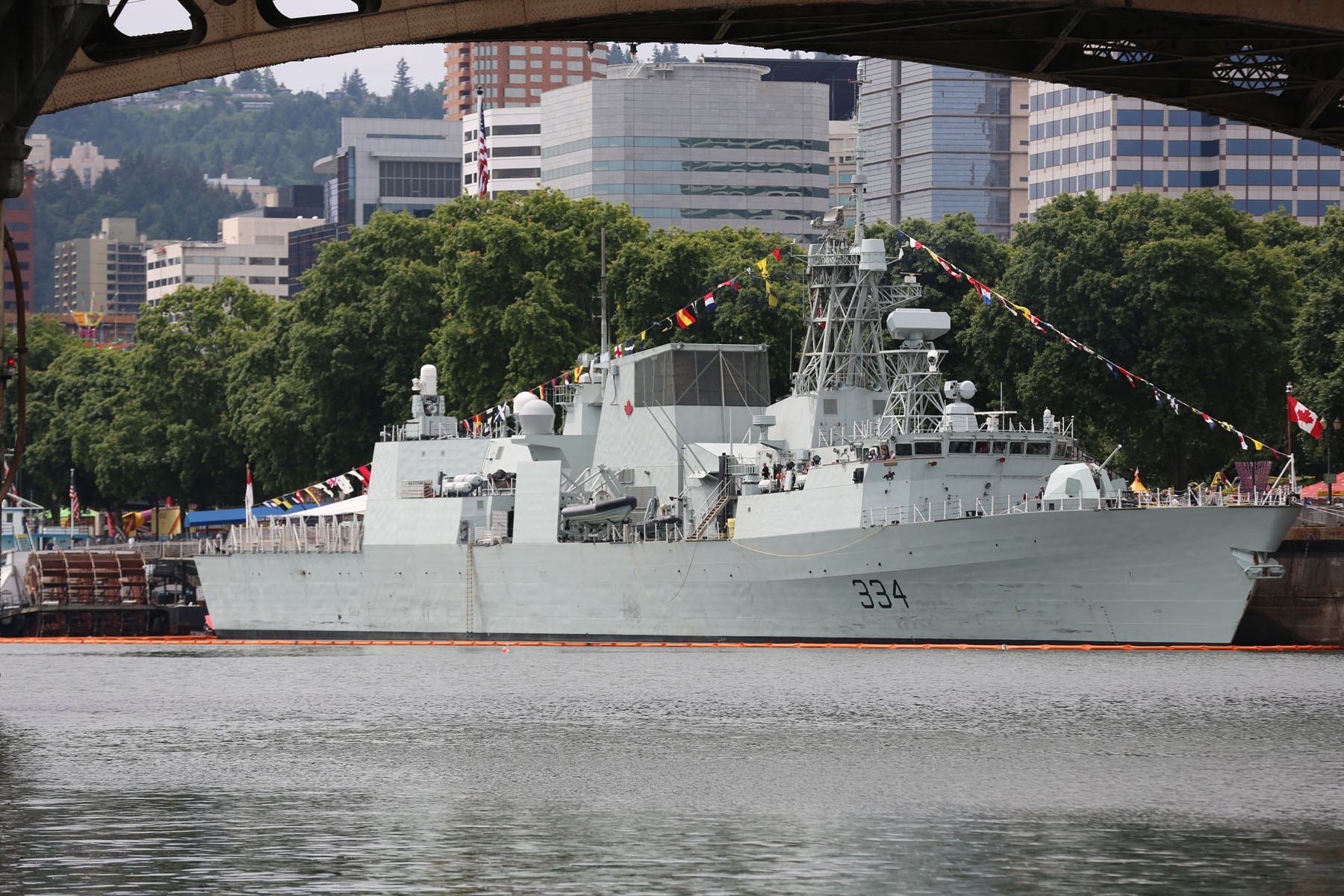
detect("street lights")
[1320,417,1341,507]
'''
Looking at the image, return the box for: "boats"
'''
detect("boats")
[191,176,1303,644]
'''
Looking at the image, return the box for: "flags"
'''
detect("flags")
[704,292,717,315]
[69,481,80,520]
[285,464,370,511]
[460,317,676,436]
[4,463,17,503]
[245,472,254,505]
[813,290,826,329]
[263,501,277,510]
[272,496,293,512]
[676,306,697,330]
[717,248,782,307]
[896,229,1280,459]
[1288,395,1323,440]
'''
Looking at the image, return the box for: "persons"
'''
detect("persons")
[20,511,223,555]
[761,462,769,479]
[1216,478,1225,492]
[1145,488,1170,506]
[773,448,896,480]
[1303,497,1311,520]
[1190,485,1198,505]
[1038,485,1045,499]
